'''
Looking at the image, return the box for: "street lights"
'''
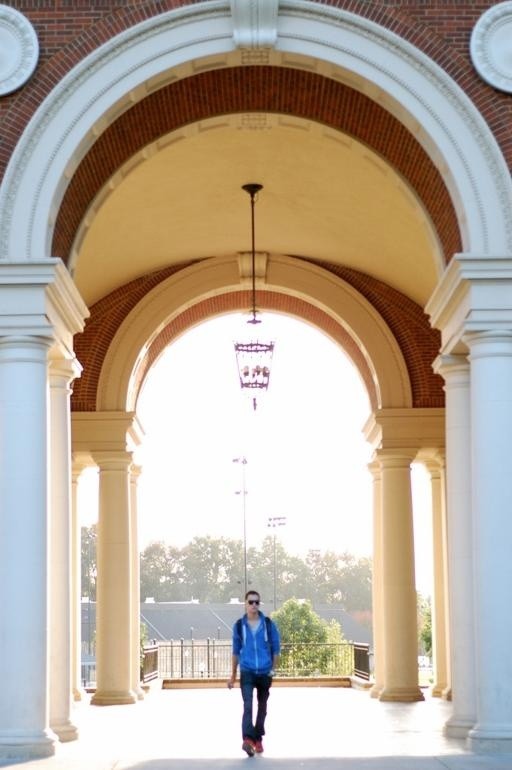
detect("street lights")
[267,516,286,610]
[85,533,97,680]
[232,456,248,594]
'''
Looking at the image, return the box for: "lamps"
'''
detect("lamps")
[234,182,274,411]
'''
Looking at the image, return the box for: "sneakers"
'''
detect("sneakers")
[255,741,264,753]
[242,739,255,756]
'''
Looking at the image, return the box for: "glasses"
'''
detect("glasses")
[248,600,259,605]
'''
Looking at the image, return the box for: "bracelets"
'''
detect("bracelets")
[269,671,276,676]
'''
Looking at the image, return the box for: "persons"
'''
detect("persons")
[225,588,281,759]
